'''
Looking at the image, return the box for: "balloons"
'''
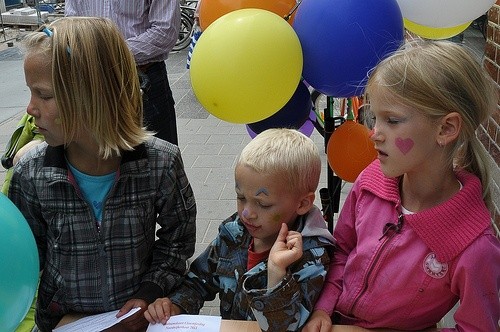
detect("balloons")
[327,121,377,183]
[189,8,303,124]
[0,193,39,332]
[199,0,498,139]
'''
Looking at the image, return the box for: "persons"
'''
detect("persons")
[64,0,181,147]
[144,129,337,332]
[305,40,500,332]
[8,17,196,332]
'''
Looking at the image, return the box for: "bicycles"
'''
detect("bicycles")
[312,90,365,132]
[169,1,198,51]
